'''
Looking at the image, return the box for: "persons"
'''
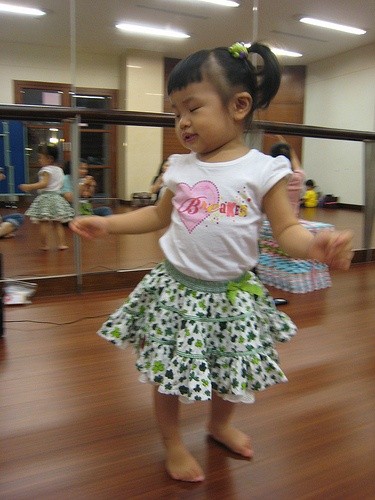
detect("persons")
[269,135,319,218]
[67,42,354,481]
[150,158,170,205]
[0,143,111,251]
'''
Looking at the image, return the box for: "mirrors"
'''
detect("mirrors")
[0,0,375,279]
